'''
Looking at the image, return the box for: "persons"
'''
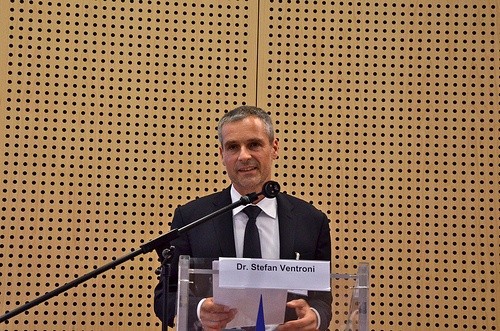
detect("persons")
[153,106,333,331]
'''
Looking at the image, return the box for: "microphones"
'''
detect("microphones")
[262,180,281,199]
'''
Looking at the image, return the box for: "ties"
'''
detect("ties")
[241,205,263,331]
